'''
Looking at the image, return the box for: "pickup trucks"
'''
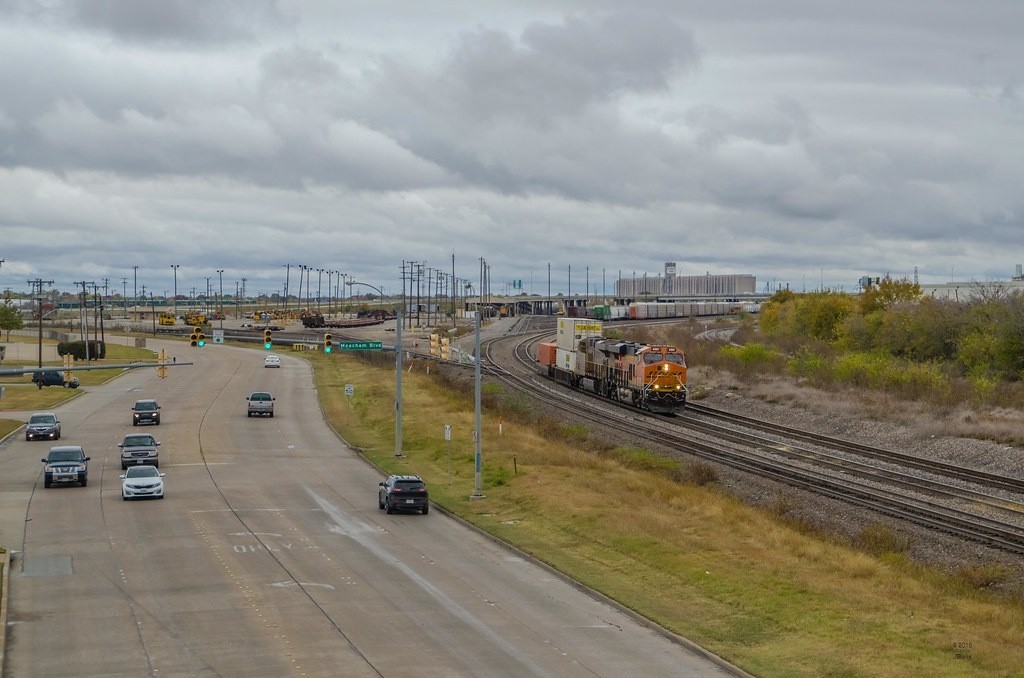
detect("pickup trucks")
[245,391,275,417]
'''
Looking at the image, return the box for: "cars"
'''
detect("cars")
[264,355,281,368]
[118,465,167,501]
[117,432,163,470]
[23,413,62,442]
[131,399,161,426]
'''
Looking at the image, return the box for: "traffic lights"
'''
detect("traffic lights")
[430,334,439,356]
[439,337,449,361]
[324,333,333,354]
[189,327,206,347]
[264,330,272,350]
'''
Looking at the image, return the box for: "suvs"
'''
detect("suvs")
[378,474,429,516]
[31,370,81,389]
[40,446,90,488]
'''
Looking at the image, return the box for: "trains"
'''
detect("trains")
[535,315,687,415]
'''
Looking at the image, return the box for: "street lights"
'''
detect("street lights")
[282,263,354,321]
[204,277,212,314]
[345,281,403,457]
[462,282,487,499]
[241,277,248,300]
[217,270,224,314]
[170,264,180,320]
[131,265,139,320]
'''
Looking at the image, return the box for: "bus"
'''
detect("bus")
[254,308,304,320]
[159,312,175,325]
[185,312,207,324]
